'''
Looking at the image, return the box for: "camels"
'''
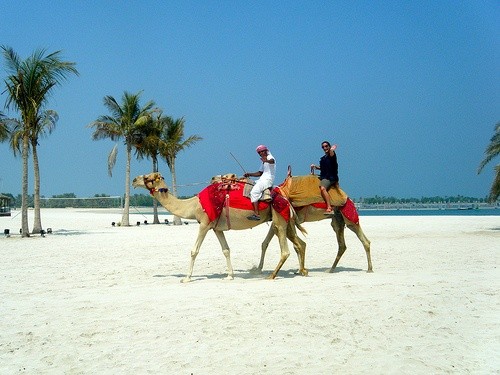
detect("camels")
[255,174,375,274]
[130,171,309,284]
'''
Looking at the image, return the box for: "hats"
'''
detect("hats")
[256,145,268,153]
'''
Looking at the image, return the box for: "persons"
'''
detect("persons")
[310,141,339,215]
[243,144,276,221]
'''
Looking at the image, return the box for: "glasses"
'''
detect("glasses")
[322,144,330,149]
[257,150,267,155]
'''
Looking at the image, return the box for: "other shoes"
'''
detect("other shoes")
[247,214,260,220]
[325,207,333,212]
